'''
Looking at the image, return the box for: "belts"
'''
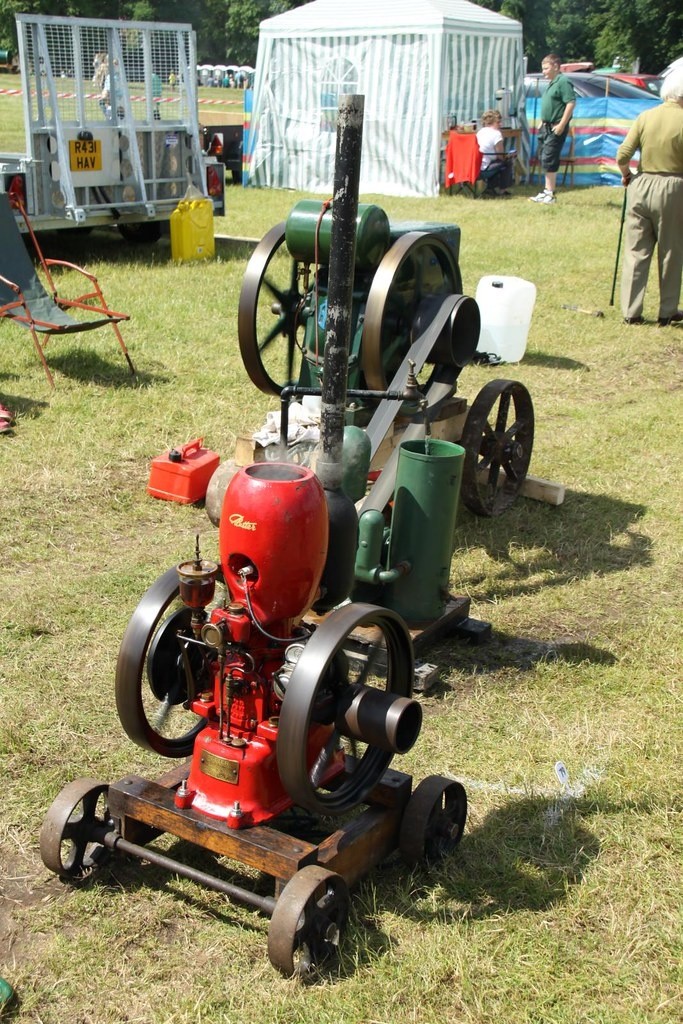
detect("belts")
[543,121,559,126]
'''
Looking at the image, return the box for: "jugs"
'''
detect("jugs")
[495,88,512,127]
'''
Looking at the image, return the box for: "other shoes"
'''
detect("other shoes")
[0,420,11,433]
[624,317,644,324]
[0,403,13,421]
[504,191,512,196]
[658,317,683,325]
[529,190,555,204]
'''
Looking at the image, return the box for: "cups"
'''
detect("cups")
[445,115,457,131]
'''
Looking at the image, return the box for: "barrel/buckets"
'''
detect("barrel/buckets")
[475,275,535,362]
[170,200,215,259]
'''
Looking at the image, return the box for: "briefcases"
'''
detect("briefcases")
[147,437,220,504]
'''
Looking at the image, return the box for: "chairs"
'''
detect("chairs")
[530,124,577,191]
[445,130,517,199]
[0,190,136,392]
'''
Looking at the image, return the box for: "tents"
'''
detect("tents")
[243,0,524,198]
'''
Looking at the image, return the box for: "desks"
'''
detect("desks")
[441,125,524,186]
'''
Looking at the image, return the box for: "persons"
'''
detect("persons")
[475,110,516,199]
[92,53,125,126]
[206,70,249,89]
[528,53,576,203]
[169,70,176,91]
[151,73,163,120]
[616,71,683,324]
